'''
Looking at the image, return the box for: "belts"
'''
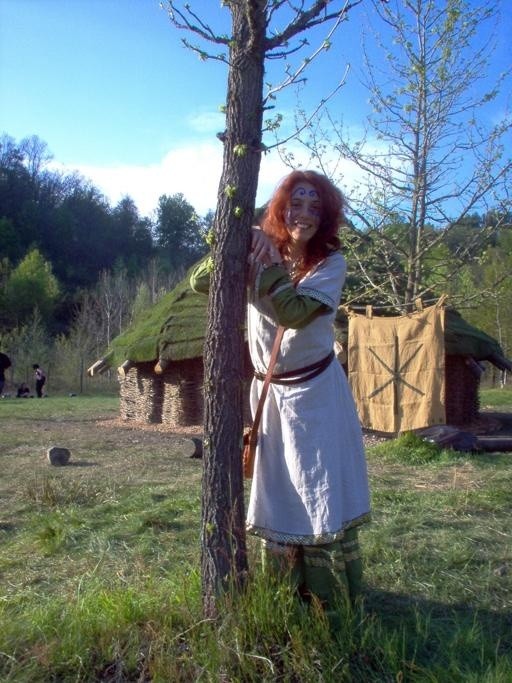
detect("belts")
[253,351,334,385]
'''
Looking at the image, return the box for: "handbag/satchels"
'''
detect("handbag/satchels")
[243,426,258,479]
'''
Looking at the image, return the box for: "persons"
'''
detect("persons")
[32,364,46,398]
[17,383,30,397]
[0,352,12,395]
[188,169,373,619]
